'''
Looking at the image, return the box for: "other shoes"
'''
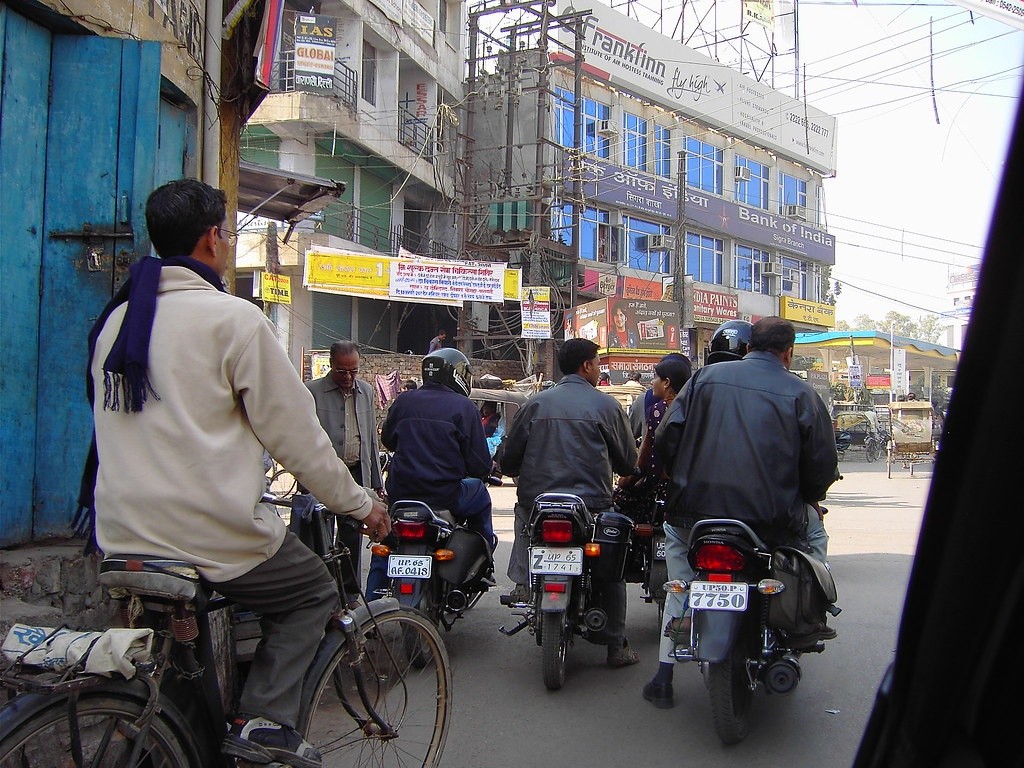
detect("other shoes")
[366,629,379,641]
[341,593,362,610]
[510,583,529,601]
[606,637,640,668]
[481,573,497,586]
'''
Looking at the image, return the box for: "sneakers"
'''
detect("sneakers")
[220,712,322,768]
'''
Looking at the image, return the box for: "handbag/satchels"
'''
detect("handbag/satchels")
[611,486,642,517]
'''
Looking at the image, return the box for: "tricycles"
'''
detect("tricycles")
[887,401,940,475]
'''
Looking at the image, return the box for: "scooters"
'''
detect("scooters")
[640,503,683,644]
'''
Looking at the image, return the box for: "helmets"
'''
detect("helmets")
[704,320,753,365]
[421,347,474,398]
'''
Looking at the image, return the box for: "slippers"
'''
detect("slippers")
[792,623,836,646]
[665,617,689,644]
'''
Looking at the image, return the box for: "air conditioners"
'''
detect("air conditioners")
[649,234,676,250]
[599,119,617,135]
[786,205,806,221]
[763,262,783,276]
[735,165,751,182]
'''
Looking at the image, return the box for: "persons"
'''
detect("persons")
[398,378,420,396]
[297,340,387,612]
[429,330,447,355]
[619,315,837,709]
[597,370,645,389]
[610,302,637,349]
[898,392,919,402]
[500,340,640,669]
[93,178,392,768]
[365,348,497,633]
[565,313,576,341]
[473,395,505,453]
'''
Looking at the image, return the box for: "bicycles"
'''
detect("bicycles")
[1,489,452,768]
[834,421,891,463]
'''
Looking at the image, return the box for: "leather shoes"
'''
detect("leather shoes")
[642,679,675,709]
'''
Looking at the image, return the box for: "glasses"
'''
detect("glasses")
[218,228,240,247]
[335,369,359,375]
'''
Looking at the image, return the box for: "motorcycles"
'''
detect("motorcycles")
[666,472,844,742]
[375,478,507,667]
[497,492,634,693]
[469,389,527,486]
